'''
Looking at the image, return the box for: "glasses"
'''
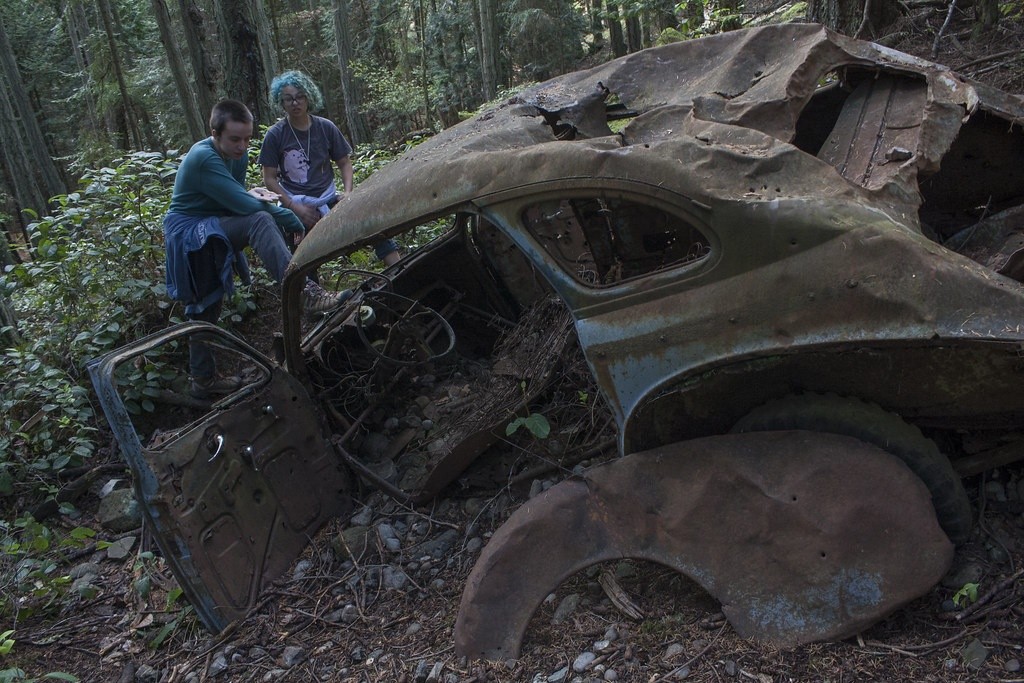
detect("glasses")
[281,93,308,106]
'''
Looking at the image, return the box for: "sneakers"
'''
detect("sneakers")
[190,373,243,397]
[299,279,351,323]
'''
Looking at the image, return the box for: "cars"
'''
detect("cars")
[82,20,1024,632]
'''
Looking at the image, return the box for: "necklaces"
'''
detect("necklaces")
[287,115,310,165]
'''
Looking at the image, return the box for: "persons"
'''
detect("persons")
[258,71,401,286]
[164,100,353,399]
